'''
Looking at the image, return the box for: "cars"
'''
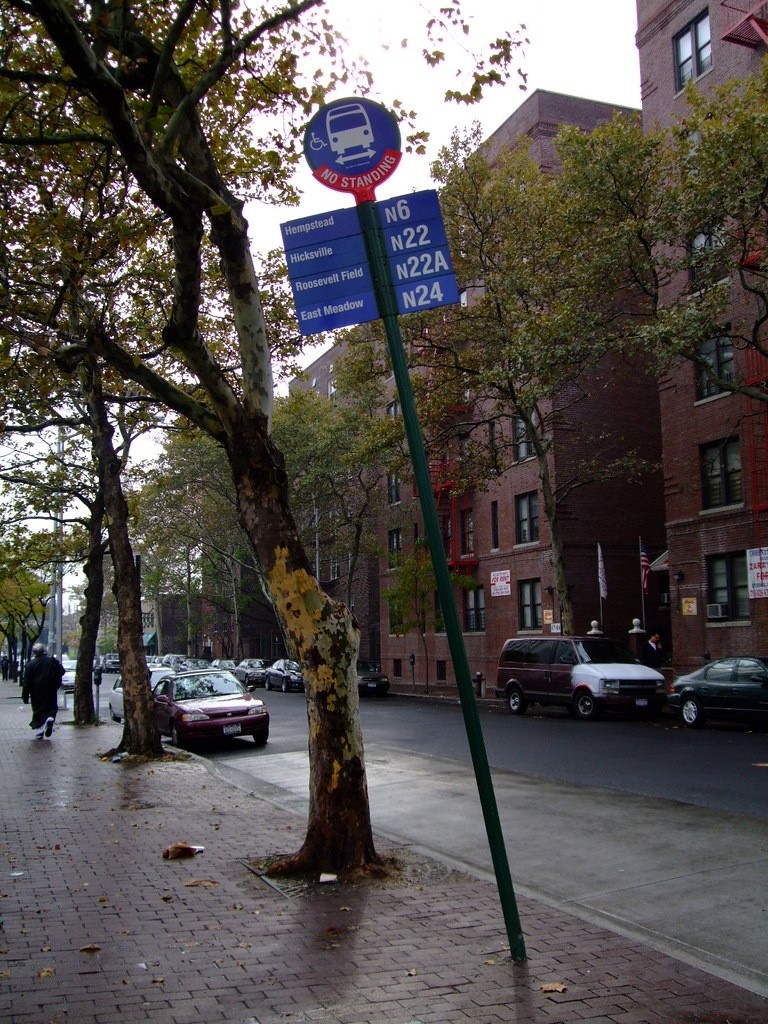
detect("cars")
[92,654,238,675]
[265,659,304,693]
[152,668,270,748]
[107,668,178,724]
[666,655,768,729]
[356,660,390,696]
[53,653,79,694]
[233,658,273,685]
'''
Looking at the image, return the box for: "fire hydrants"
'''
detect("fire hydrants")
[472,670,482,699]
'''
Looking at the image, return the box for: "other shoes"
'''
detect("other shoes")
[36,733,43,739]
[45,720,53,737]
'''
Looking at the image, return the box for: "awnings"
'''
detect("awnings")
[142,631,158,646]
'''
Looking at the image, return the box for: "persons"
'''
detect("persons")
[642,631,664,671]
[0,656,9,681]
[22,643,66,739]
[12,659,19,683]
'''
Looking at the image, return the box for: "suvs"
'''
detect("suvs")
[494,634,669,721]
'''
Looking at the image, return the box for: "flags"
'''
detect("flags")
[598,547,608,598]
[639,541,650,593]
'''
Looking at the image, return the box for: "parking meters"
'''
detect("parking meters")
[94,667,102,725]
[409,652,416,693]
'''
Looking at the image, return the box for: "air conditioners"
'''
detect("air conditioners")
[706,604,727,618]
[661,593,670,603]
